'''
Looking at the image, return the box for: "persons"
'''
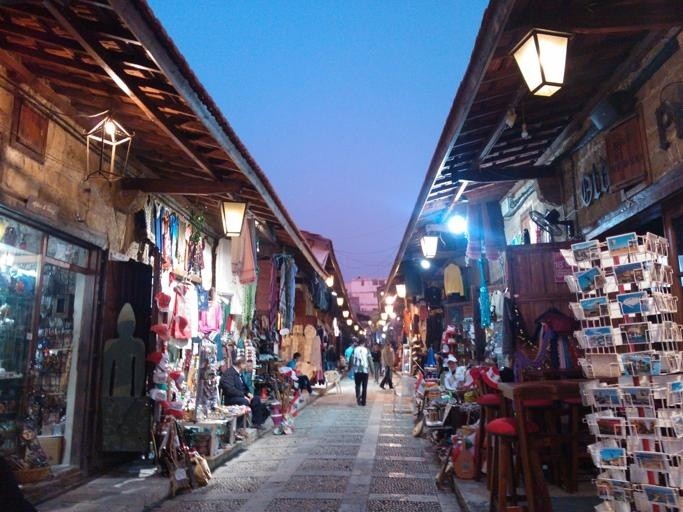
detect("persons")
[284,351,320,402]
[343,339,376,406]
[429,356,467,411]
[320,335,401,391]
[218,354,268,431]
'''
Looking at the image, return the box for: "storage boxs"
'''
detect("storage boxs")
[25,434,62,466]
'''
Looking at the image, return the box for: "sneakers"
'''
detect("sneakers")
[358,401,366,405]
[380,383,393,388]
[299,395,304,403]
[310,391,320,396]
[251,423,266,430]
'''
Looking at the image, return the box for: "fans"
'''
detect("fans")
[528,208,573,238]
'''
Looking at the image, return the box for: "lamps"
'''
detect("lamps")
[420,235,440,258]
[216,192,252,236]
[82,95,136,191]
[508,25,573,99]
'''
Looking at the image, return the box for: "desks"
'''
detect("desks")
[180,408,246,457]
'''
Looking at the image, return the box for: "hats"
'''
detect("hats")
[149,388,170,407]
[169,370,185,390]
[154,371,169,390]
[447,356,458,364]
[147,351,163,365]
[481,367,500,389]
[154,292,172,313]
[442,345,449,353]
[150,324,168,339]
[357,337,367,344]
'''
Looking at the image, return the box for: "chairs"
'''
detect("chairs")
[469,363,599,512]
